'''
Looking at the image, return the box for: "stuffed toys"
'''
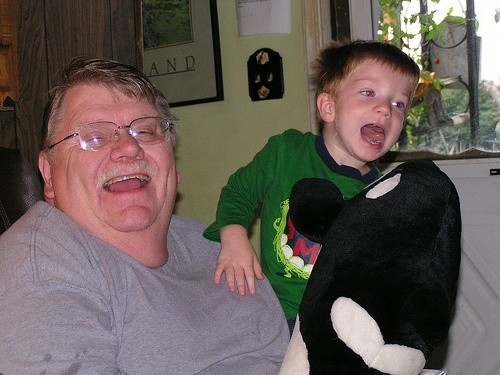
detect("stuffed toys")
[276,157,463,375]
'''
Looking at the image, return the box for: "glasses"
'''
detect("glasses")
[49,116,175,151]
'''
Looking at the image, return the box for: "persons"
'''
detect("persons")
[202,38,421,342]
[0,56,291,375]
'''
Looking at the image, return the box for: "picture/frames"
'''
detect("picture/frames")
[142,0,224,108]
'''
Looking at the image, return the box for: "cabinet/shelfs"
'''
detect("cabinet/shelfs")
[0,0,21,112]
